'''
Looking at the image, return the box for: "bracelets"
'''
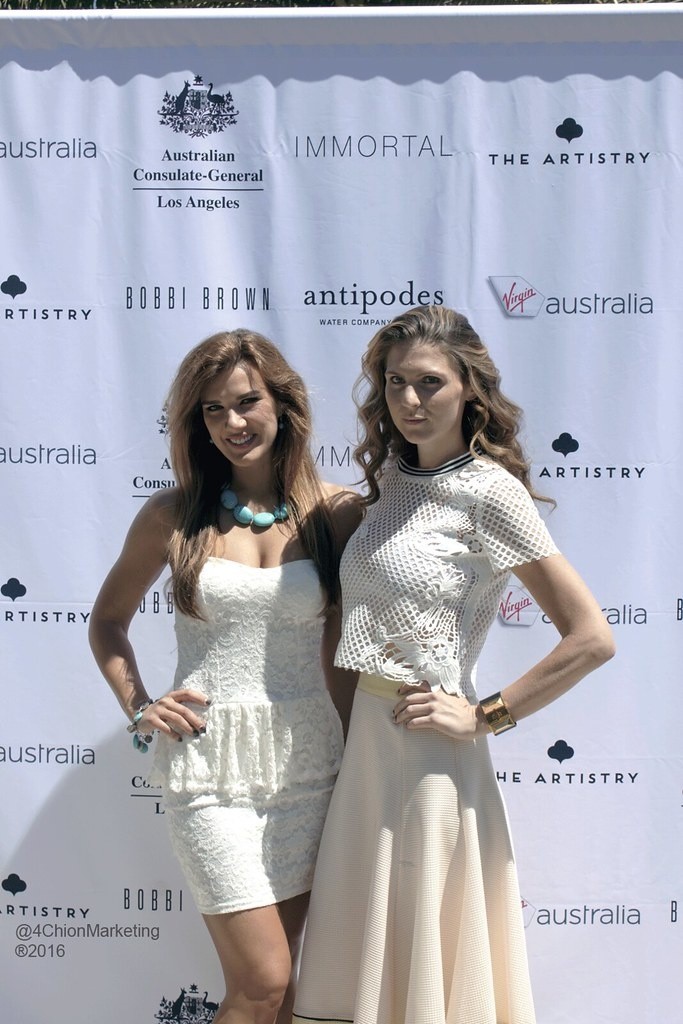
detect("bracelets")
[479,692,517,735]
[127,699,161,753]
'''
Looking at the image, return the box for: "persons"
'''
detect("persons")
[292,304,616,1024]
[89,329,366,1024]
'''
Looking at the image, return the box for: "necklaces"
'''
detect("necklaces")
[221,484,288,527]
